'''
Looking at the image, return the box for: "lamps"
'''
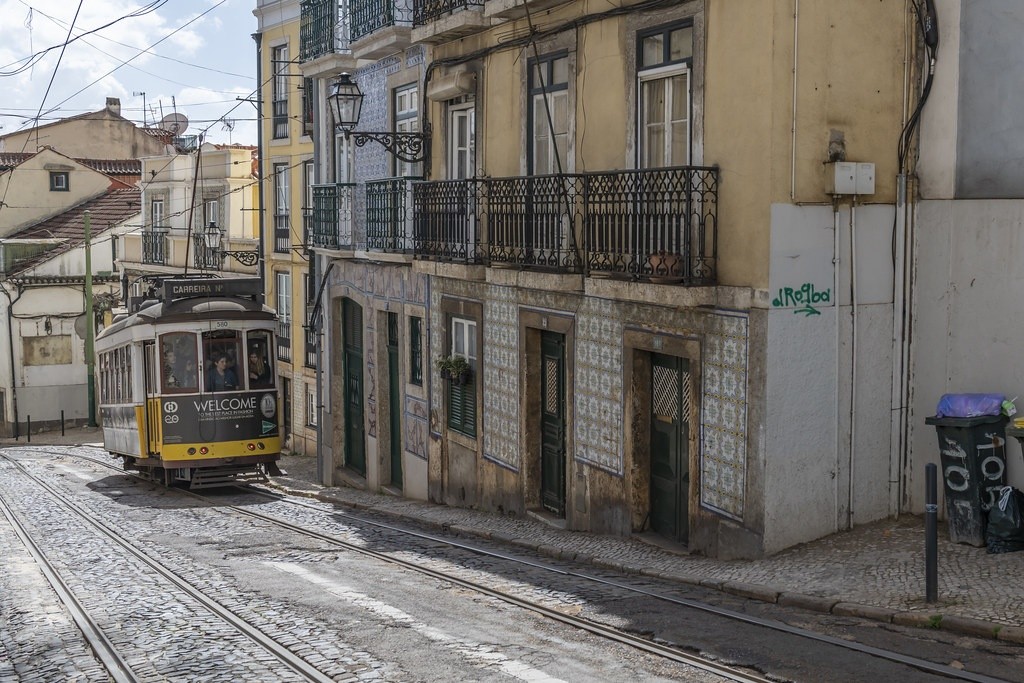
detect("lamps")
[201,220,260,266]
[326,71,431,175]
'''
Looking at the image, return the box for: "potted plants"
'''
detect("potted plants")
[433,354,471,385]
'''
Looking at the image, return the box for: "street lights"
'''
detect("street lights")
[133,91,146,129]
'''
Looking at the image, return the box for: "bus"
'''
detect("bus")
[94,135,288,491]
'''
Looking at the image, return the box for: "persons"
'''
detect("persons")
[249,349,270,382]
[210,348,221,359]
[164,350,197,388]
[206,353,238,391]
[226,348,235,369]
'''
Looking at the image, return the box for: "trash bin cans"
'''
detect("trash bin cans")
[925,415,1012,548]
